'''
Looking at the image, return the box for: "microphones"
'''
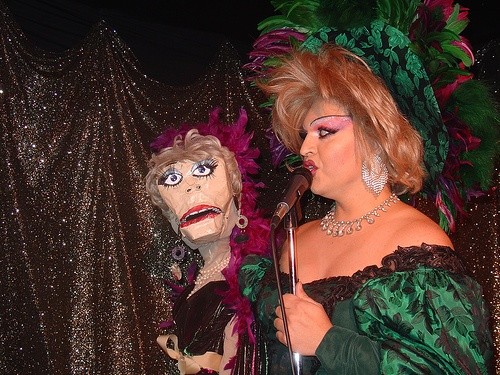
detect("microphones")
[270,168,313,229]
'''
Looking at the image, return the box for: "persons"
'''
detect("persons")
[238,0,500,375]
[145,107,288,375]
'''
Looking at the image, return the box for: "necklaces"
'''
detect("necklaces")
[320,192,400,237]
[187,258,230,299]
